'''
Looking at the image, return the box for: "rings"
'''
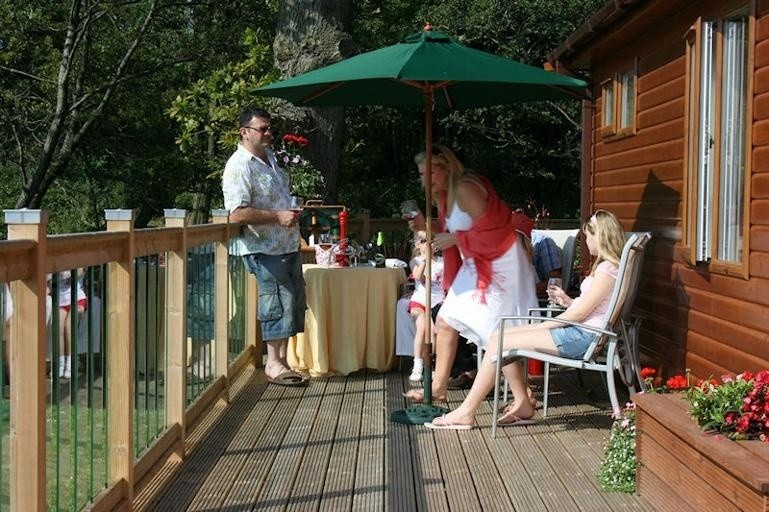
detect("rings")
[435,247,438,251]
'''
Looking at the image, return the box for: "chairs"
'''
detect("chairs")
[527,227,580,299]
[491,232,653,434]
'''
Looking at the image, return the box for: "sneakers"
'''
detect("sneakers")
[410,366,423,381]
[448,374,473,390]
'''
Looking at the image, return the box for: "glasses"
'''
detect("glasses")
[590,208,603,223]
[244,127,269,134]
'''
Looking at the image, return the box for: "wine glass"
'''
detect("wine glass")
[547,277,563,309]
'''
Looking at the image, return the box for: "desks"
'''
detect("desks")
[288,261,399,382]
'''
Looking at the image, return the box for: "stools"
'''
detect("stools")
[394,295,419,373]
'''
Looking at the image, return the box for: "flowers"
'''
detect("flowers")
[688,357,769,448]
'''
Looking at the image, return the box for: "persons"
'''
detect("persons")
[401,144,544,414]
[222,103,311,387]
[408,227,446,382]
[423,209,628,430]
[444,236,563,398]
[45,266,88,379]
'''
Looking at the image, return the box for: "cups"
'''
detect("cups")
[290,195,305,214]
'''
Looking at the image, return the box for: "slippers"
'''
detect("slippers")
[402,391,446,403]
[267,372,307,386]
[498,413,538,426]
[424,413,473,430]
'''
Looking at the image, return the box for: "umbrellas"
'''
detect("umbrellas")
[248,21,595,406]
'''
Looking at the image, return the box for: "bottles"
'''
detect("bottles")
[376,231,386,268]
